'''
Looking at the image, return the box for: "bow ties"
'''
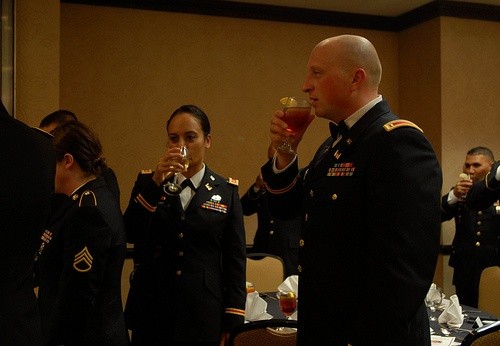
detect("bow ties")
[177,179,197,195]
[328,120,349,141]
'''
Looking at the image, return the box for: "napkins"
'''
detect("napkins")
[431,333,460,346]
[426,283,449,311]
[277,275,299,300]
[242,288,272,321]
[438,294,465,332]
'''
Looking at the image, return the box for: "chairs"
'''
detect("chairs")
[460,323,500,346]
[246,248,285,293]
[230,315,301,346]
[476,266,500,319]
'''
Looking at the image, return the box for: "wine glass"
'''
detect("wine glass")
[163,146,190,197]
[273,96,312,155]
[274,291,296,332]
[458,173,474,202]
[425,288,443,322]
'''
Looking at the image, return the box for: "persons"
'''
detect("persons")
[0,99,58,346]
[260,34,443,346]
[442,147,500,309]
[241,142,301,276]
[122,104,247,346]
[30,109,127,346]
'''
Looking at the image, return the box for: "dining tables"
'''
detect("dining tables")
[244,284,500,346]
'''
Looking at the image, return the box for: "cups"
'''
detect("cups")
[246,281,256,293]
